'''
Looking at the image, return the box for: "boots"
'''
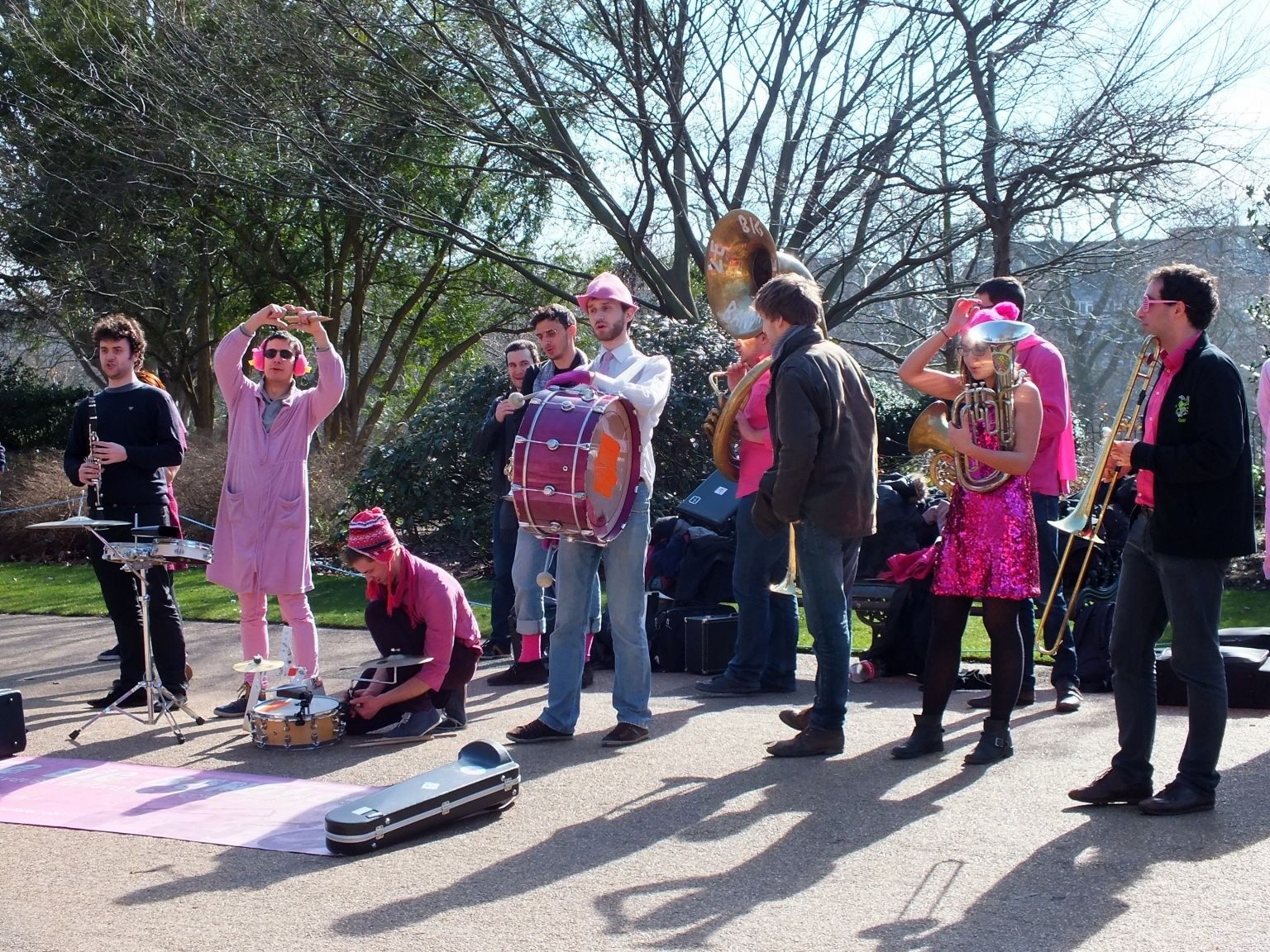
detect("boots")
[963,719,1015,765]
[891,713,944,758]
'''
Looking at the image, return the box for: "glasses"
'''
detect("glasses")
[1142,293,1191,314]
[263,348,298,360]
[536,326,563,342]
[957,345,990,357]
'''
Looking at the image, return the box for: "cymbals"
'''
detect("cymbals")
[232,659,285,673]
[24,517,134,530]
[357,655,435,669]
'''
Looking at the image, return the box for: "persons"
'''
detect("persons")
[476,340,540,660]
[506,272,673,745]
[968,278,1081,711]
[891,298,1043,764]
[487,304,602,687]
[340,507,483,737]
[62,312,188,712]
[1067,263,1257,814]
[695,332,800,697]
[752,273,878,757]
[206,303,347,716]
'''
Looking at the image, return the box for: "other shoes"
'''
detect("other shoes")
[1054,680,1083,711]
[97,642,121,660]
[505,719,572,743]
[766,711,844,757]
[384,688,467,739]
[154,691,188,712]
[486,660,547,687]
[214,696,264,718]
[695,670,799,694]
[966,690,1035,707]
[601,722,650,746]
[87,684,147,707]
[581,662,593,689]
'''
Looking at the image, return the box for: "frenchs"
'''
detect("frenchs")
[701,206,831,483]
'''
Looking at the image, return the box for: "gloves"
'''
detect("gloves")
[545,370,591,389]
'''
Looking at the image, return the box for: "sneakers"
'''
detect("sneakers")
[1069,767,1216,813]
[481,637,511,658]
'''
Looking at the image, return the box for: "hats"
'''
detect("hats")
[346,506,406,618]
[958,301,1020,339]
[576,272,639,313]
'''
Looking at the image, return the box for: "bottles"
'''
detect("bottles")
[849,659,877,683]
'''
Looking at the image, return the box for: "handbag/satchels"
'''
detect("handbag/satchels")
[653,604,691,672]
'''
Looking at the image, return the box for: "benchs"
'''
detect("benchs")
[851,574,1123,649]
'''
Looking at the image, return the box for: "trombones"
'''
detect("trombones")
[1033,332,1163,657]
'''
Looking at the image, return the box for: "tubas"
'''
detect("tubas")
[949,319,1037,494]
[906,400,959,502]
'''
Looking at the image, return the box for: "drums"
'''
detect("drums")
[506,384,644,549]
[149,537,215,566]
[248,694,344,750]
[101,542,173,566]
